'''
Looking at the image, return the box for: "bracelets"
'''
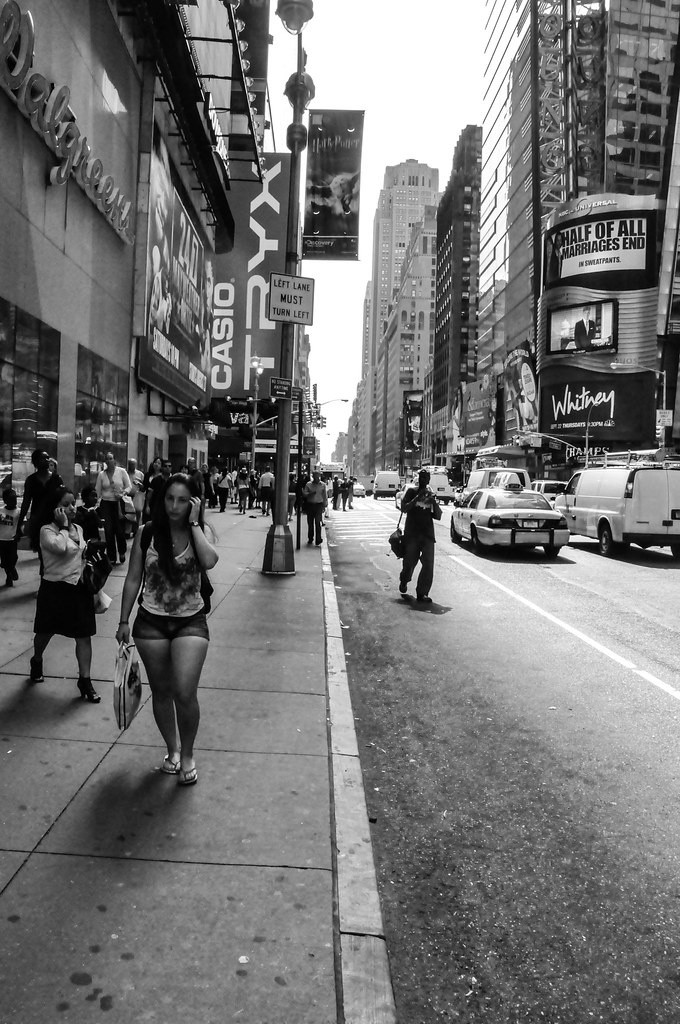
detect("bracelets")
[119,621,129,625]
[59,526,69,531]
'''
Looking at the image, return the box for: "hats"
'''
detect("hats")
[240,467,248,475]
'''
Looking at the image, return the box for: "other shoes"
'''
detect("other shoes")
[399,580,407,593]
[4,579,15,587]
[220,508,225,512]
[239,507,243,512]
[323,523,326,527]
[416,594,432,603]
[12,568,19,580]
[308,539,314,544]
[243,511,246,514]
[208,504,216,509]
[248,505,262,509]
[263,510,270,516]
[120,552,126,565]
[315,538,323,545]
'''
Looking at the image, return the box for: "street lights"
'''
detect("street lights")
[442,417,468,484]
[610,362,667,450]
[248,351,265,470]
[584,402,601,468]
[260,0,315,578]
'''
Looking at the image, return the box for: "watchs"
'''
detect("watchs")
[190,521,198,526]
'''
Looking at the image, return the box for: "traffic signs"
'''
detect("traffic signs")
[303,436,316,459]
[291,386,304,402]
[269,376,293,401]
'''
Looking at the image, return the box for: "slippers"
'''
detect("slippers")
[163,754,181,776]
[180,767,198,787]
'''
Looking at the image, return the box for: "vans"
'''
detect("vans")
[454,466,533,508]
[550,450,680,562]
[411,465,456,505]
[370,471,402,500]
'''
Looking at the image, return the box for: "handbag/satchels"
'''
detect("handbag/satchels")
[93,590,113,615]
[113,640,143,729]
[117,495,137,525]
[84,543,113,594]
[387,528,408,559]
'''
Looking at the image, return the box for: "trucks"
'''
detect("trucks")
[317,461,374,498]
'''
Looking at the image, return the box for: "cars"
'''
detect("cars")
[449,471,571,558]
[530,479,569,508]
[395,483,436,512]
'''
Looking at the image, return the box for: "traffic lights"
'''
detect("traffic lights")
[319,414,327,429]
[315,411,321,428]
[316,439,320,451]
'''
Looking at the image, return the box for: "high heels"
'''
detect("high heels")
[30,656,45,682]
[75,676,102,703]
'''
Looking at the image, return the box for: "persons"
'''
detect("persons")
[545,232,563,282]
[574,306,596,349]
[0,449,356,589]
[398,469,442,603]
[116,473,219,786]
[30,486,102,702]
